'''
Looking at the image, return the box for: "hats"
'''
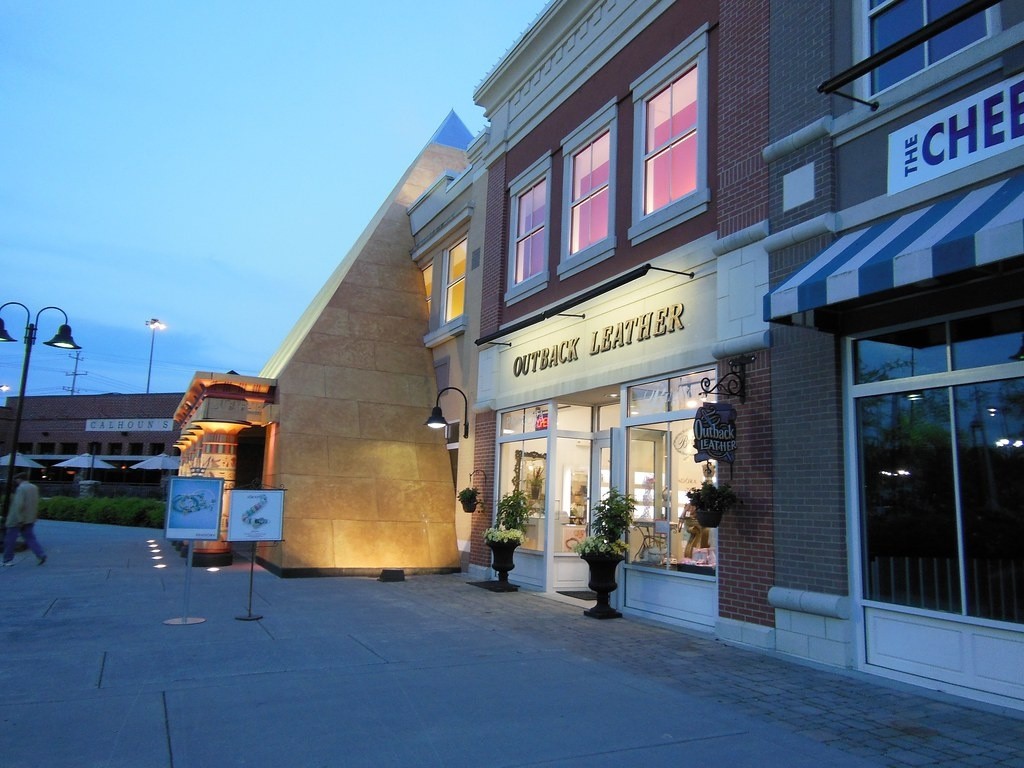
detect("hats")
[12,472,27,479]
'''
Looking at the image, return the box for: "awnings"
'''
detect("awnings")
[761,176,1024,336]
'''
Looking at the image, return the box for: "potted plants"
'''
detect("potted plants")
[686,482,740,527]
[457,487,480,512]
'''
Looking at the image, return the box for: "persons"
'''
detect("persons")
[570,493,587,524]
[0,473,47,567]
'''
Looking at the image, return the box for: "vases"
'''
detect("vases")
[483,541,521,583]
[581,555,622,617]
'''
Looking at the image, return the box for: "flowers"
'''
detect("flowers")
[575,534,631,558]
[481,526,525,546]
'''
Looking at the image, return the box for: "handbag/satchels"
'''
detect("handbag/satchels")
[644,547,662,564]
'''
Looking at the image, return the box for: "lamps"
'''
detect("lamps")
[475,263,696,347]
[424,385,469,439]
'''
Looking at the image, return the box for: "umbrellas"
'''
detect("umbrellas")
[129,453,179,469]
[0,450,45,469]
[51,452,117,469]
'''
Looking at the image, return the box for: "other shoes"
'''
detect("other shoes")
[37,555,48,566]
[0,561,14,567]
